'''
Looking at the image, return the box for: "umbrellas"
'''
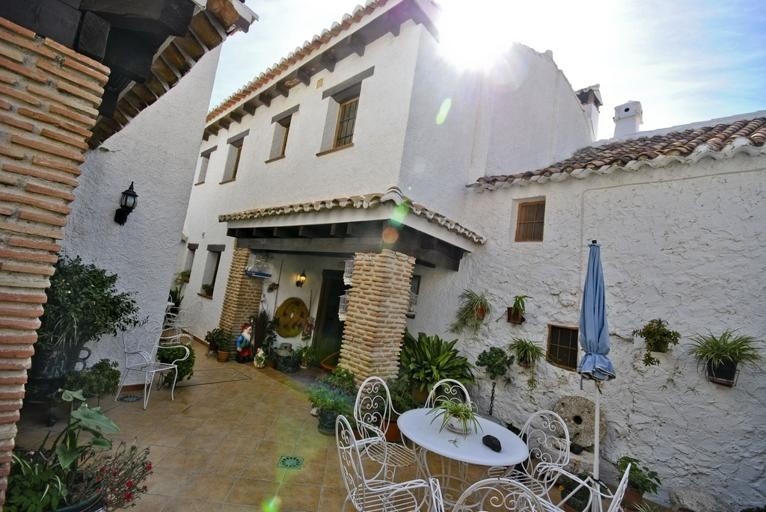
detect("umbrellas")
[576,239,618,511]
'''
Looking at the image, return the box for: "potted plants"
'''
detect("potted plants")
[616,455,661,510]
[23,251,149,405]
[496,296,533,325]
[395,326,476,406]
[205,329,223,354]
[305,382,363,435]
[633,319,682,366]
[444,288,493,338]
[689,331,765,386]
[3,387,104,512]
[216,329,233,362]
[512,342,546,405]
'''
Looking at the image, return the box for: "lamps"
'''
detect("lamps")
[114,181,138,225]
[296,270,306,288]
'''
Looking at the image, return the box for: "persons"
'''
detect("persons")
[236,322,253,363]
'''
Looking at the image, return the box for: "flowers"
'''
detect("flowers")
[67,437,153,512]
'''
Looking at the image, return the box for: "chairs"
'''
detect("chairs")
[114,295,203,411]
